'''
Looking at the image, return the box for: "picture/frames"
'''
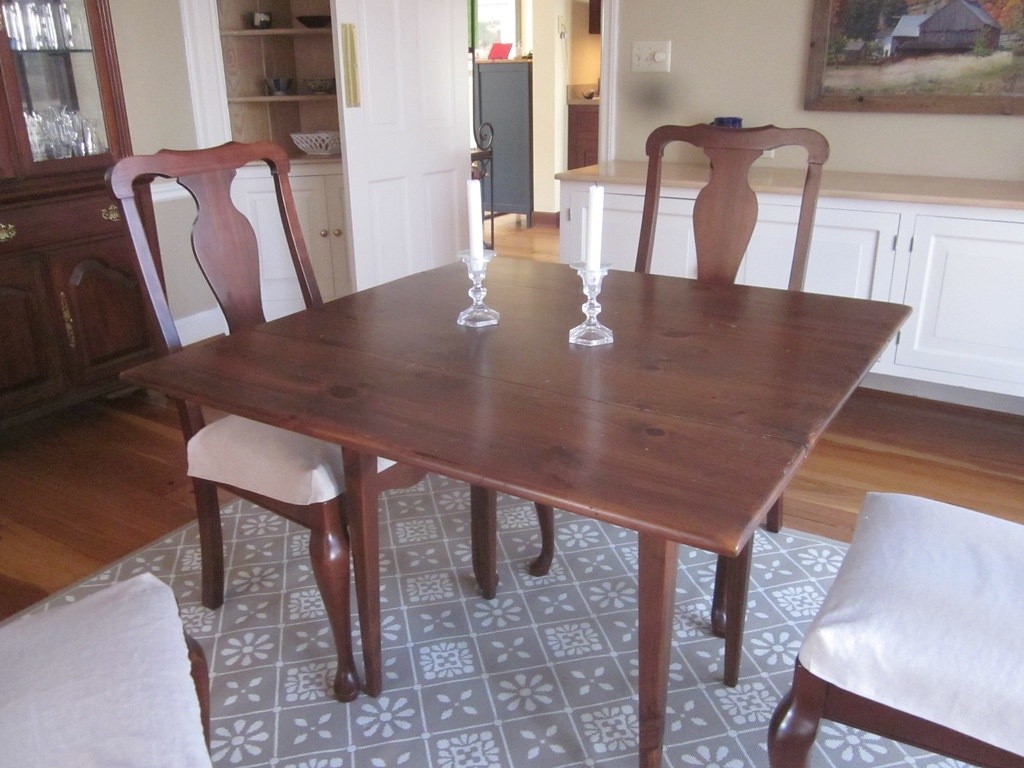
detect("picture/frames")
[802,1,1024,118]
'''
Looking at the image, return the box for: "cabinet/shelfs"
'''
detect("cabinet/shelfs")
[474,63,532,228]
[553,186,1024,416]
[568,108,597,171]
[0,0,169,436]
[218,0,352,315]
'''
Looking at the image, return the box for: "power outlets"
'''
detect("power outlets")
[631,40,673,73]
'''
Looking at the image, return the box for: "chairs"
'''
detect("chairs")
[0,572,210,768]
[109,138,476,702]
[527,120,829,636]
[767,491,1024,768]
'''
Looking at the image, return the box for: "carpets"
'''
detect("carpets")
[0,499,968,768]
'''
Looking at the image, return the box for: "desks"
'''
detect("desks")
[123,255,913,768]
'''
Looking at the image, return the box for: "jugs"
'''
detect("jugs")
[709,117,743,168]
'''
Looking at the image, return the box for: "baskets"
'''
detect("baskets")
[291,131,341,154]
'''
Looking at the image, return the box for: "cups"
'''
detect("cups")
[252,12,271,29]
[23,105,102,162]
[1,2,74,50]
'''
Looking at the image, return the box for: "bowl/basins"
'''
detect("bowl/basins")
[296,16,331,28]
[264,78,293,96]
[304,78,335,95]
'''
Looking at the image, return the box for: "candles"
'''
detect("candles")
[467,177,482,254]
[587,183,605,272]
